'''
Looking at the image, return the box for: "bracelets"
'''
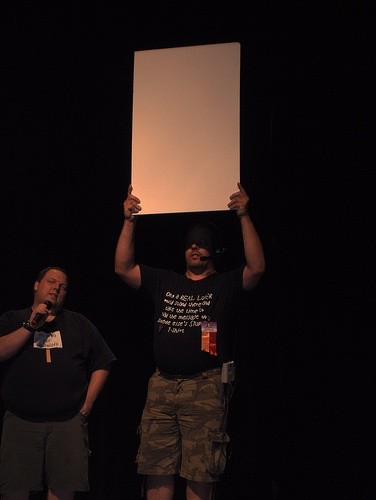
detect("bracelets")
[22,321,36,333]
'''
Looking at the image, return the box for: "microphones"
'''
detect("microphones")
[200,255,214,261]
[32,301,53,326]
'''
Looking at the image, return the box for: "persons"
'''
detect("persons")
[115,183,265,500]
[0,266,117,500]
